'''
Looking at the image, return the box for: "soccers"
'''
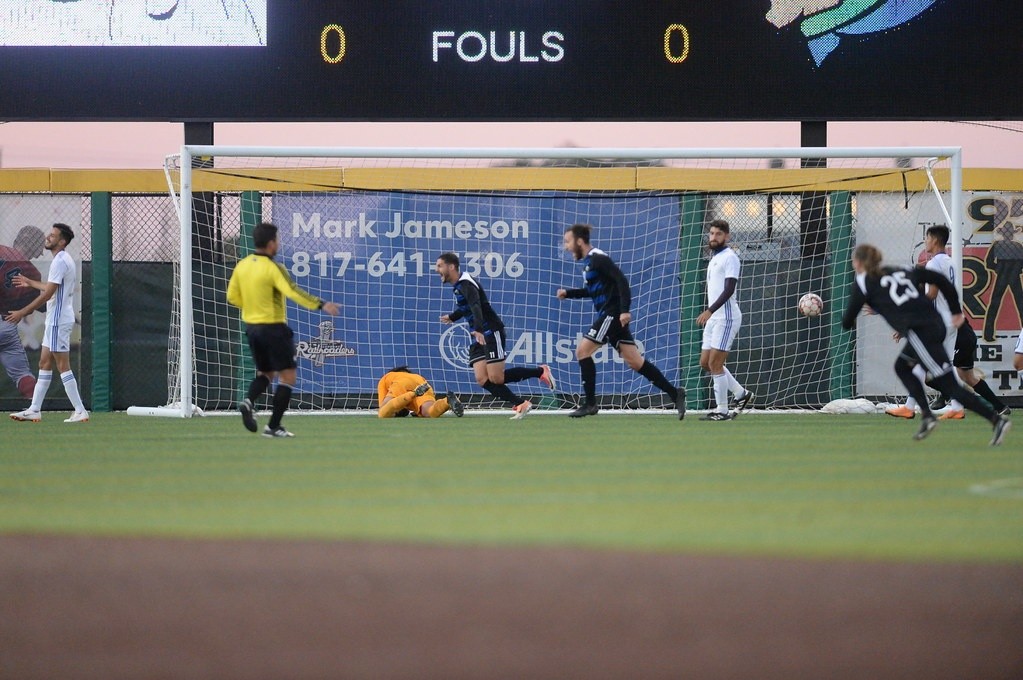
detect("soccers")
[799,293,824,318]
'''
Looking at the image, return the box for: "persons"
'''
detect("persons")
[696,220,754,421]
[436,254,556,420]
[885,224,965,419]
[227,224,341,438]
[842,244,1010,446]
[931,319,1012,416]
[5,223,89,423]
[1013,328,1023,376]
[557,225,686,420]
[378,366,464,417]
[0,225,47,399]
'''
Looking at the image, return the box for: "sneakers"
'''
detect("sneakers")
[938,410,966,419]
[261,425,295,437]
[676,388,687,420]
[63,410,90,422]
[885,405,915,419]
[731,388,754,420]
[239,398,258,432]
[931,394,953,410]
[446,390,464,417]
[698,411,731,421]
[999,405,1012,416]
[538,365,556,392]
[990,417,1013,445]
[509,400,532,420]
[569,402,598,416]
[912,415,937,439]
[9,409,41,422]
[415,383,430,396]
